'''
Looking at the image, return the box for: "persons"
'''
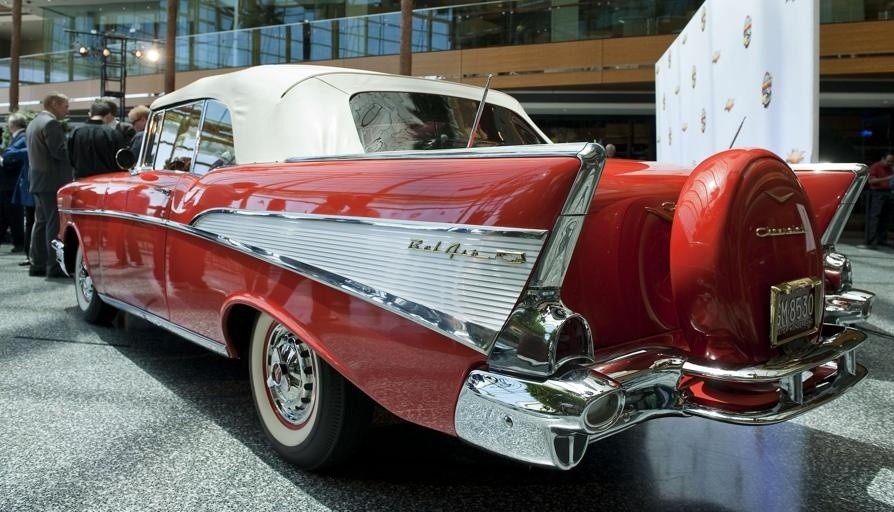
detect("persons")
[605,143,617,159]
[865,150,894,246]
[162,156,191,172]
[0,91,152,278]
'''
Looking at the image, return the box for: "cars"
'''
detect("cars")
[51,64,875,475]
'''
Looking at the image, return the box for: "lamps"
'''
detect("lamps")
[62,28,164,59]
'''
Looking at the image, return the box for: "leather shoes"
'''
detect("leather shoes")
[12,247,74,279]
